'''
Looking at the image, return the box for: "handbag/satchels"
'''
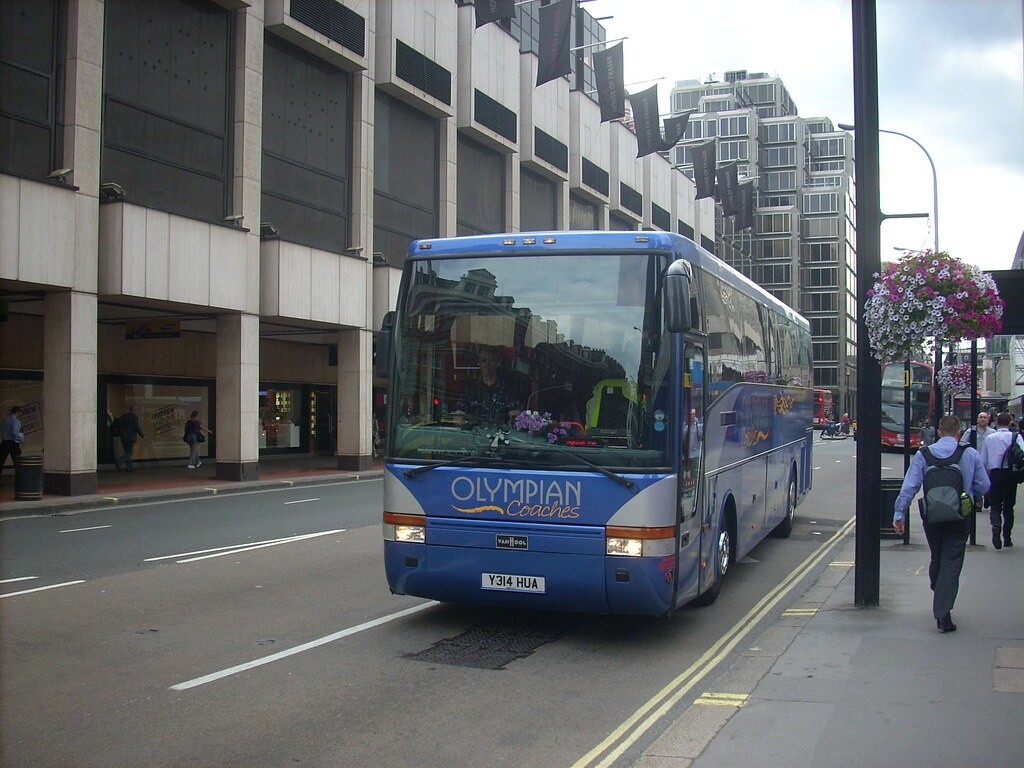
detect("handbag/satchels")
[196,432,205,444]
[112,418,122,437]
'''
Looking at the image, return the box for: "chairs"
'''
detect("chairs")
[582,378,642,449]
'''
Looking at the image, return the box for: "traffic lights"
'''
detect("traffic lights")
[434,399,440,406]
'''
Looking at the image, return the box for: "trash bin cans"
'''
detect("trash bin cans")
[881,478,904,539]
[14,456,43,500]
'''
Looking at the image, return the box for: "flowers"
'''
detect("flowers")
[861,249,1004,367]
[514,410,570,444]
[934,364,972,395]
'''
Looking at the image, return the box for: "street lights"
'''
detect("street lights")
[838,122,943,421]
[993,356,1003,392]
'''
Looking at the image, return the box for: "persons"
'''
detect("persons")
[820,415,836,437]
[840,412,850,437]
[1017,415,1024,431]
[960,411,996,512]
[682,408,703,492]
[183,411,213,469]
[830,419,835,438]
[111,404,147,473]
[1008,413,1015,431]
[893,415,991,631]
[991,414,999,430]
[452,348,520,429]
[107,409,121,472]
[918,418,935,447]
[981,413,1024,549]
[0,404,24,475]
[624,338,649,381]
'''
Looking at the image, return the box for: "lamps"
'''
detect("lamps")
[261,222,279,238]
[345,247,363,256]
[101,182,126,200]
[46,168,73,184]
[222,215,245,227]
[372,252,387,268]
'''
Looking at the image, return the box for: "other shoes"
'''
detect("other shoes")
[187,465,195,469]
[984,498,989,509]
[992,527,1002,549]
[114,459,121,470]
[1004,541,1013,547]
[126,468,132,473]
[936,612,957,631]
[196,461,202,467]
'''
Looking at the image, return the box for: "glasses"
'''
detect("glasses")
[479,358,498,363]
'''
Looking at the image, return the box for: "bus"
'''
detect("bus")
[375,229,814,616]
[881,361,936,450]
[814,390,833,430]
[954,397,1008,435]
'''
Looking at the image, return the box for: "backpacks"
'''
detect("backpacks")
[1001,432,1024,472]
[919,441,972,525]
